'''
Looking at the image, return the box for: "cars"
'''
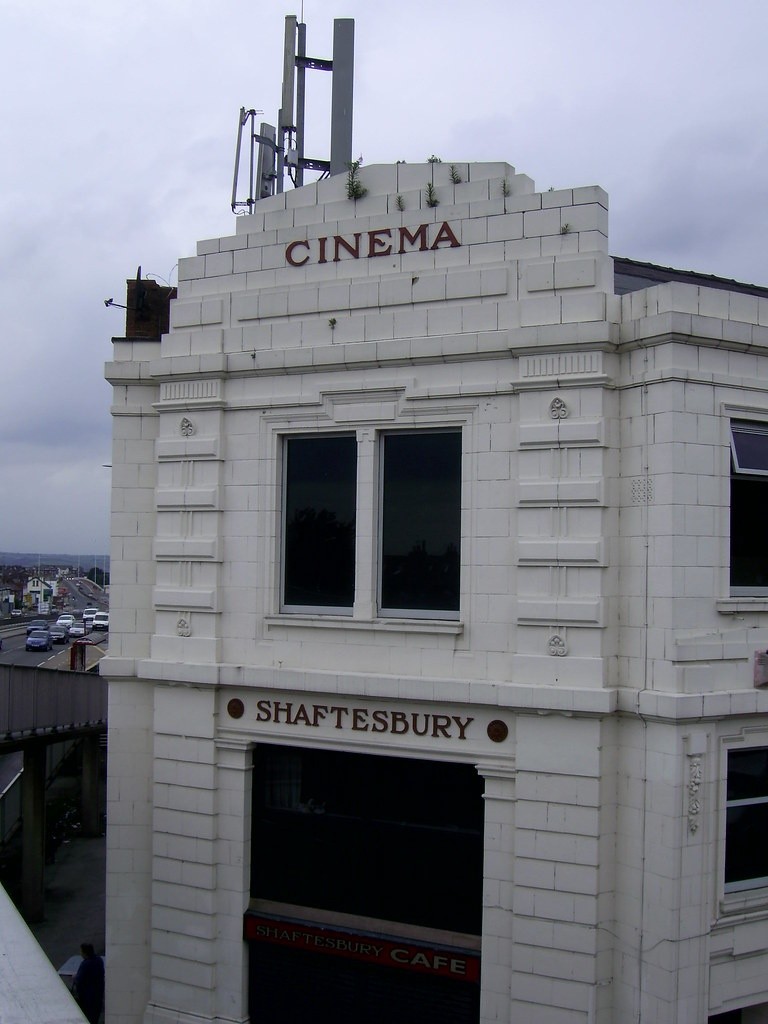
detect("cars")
[68,622,90,638]
[11,608,25,619]
[55,615,76,628]
[92,611,109,632]
[26,630,53,651]
[26,619,50,638]
[48,625,71,645]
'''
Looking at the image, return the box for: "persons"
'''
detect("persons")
[67,941,104,1024]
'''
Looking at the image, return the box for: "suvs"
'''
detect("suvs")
[83,607,99,623]
